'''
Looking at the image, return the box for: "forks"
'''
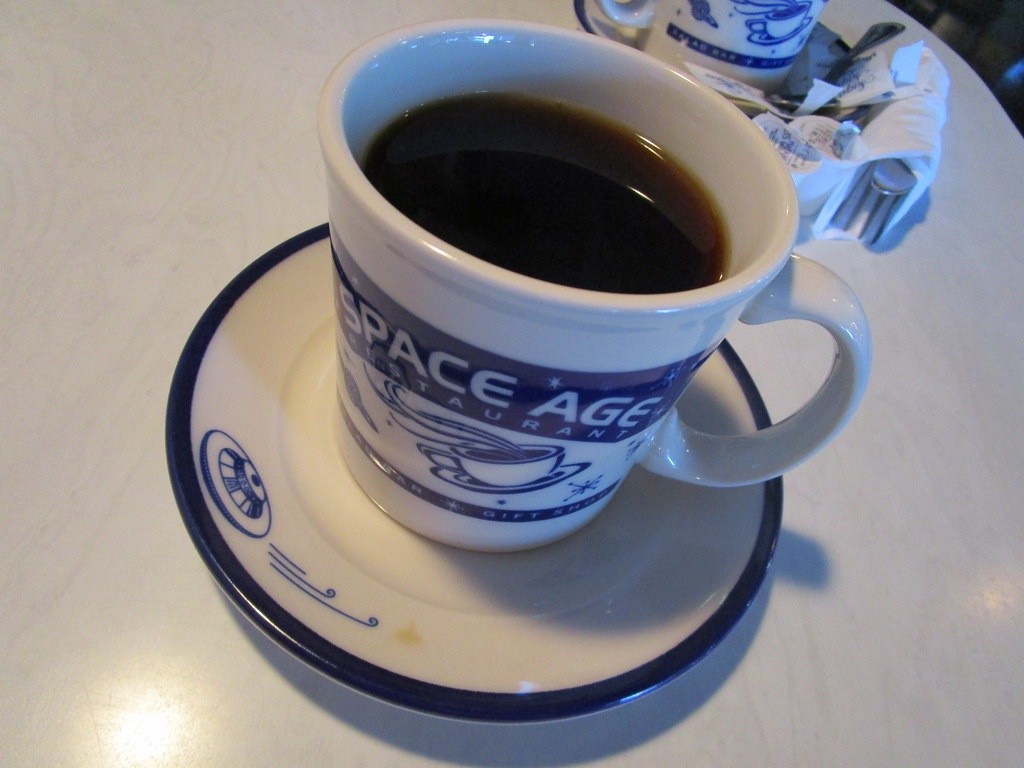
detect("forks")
[832,160,902,246]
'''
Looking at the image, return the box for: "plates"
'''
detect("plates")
[165,222,784,723]
[572,0,872,122]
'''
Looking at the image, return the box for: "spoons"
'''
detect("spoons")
[870,158,917,194]
[765,22,906,107]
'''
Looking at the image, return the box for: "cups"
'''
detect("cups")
[319,19,872,552]
[596,0,830,102]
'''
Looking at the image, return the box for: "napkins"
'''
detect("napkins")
[810,47,951,246]
